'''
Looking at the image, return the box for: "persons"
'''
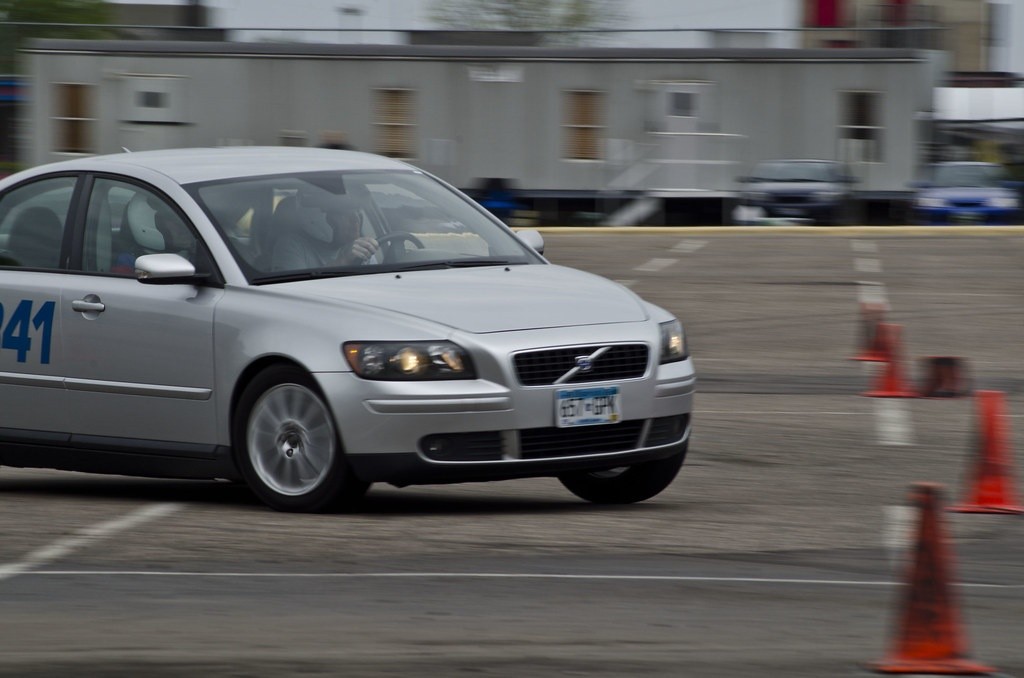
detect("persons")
[274,180,379,277]
[116,190,211,276]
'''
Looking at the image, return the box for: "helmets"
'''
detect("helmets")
[295,177,373,251]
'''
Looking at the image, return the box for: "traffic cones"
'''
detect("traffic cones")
[863,481,998,675]
[941,388,1022,515]
[860,323,921,397]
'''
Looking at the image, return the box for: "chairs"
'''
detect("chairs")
[261,197,297,272]
[7,207,63,266]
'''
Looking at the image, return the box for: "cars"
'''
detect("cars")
[908,160,1024,227]
[1,145,698,515]
[730,158,860,227]
